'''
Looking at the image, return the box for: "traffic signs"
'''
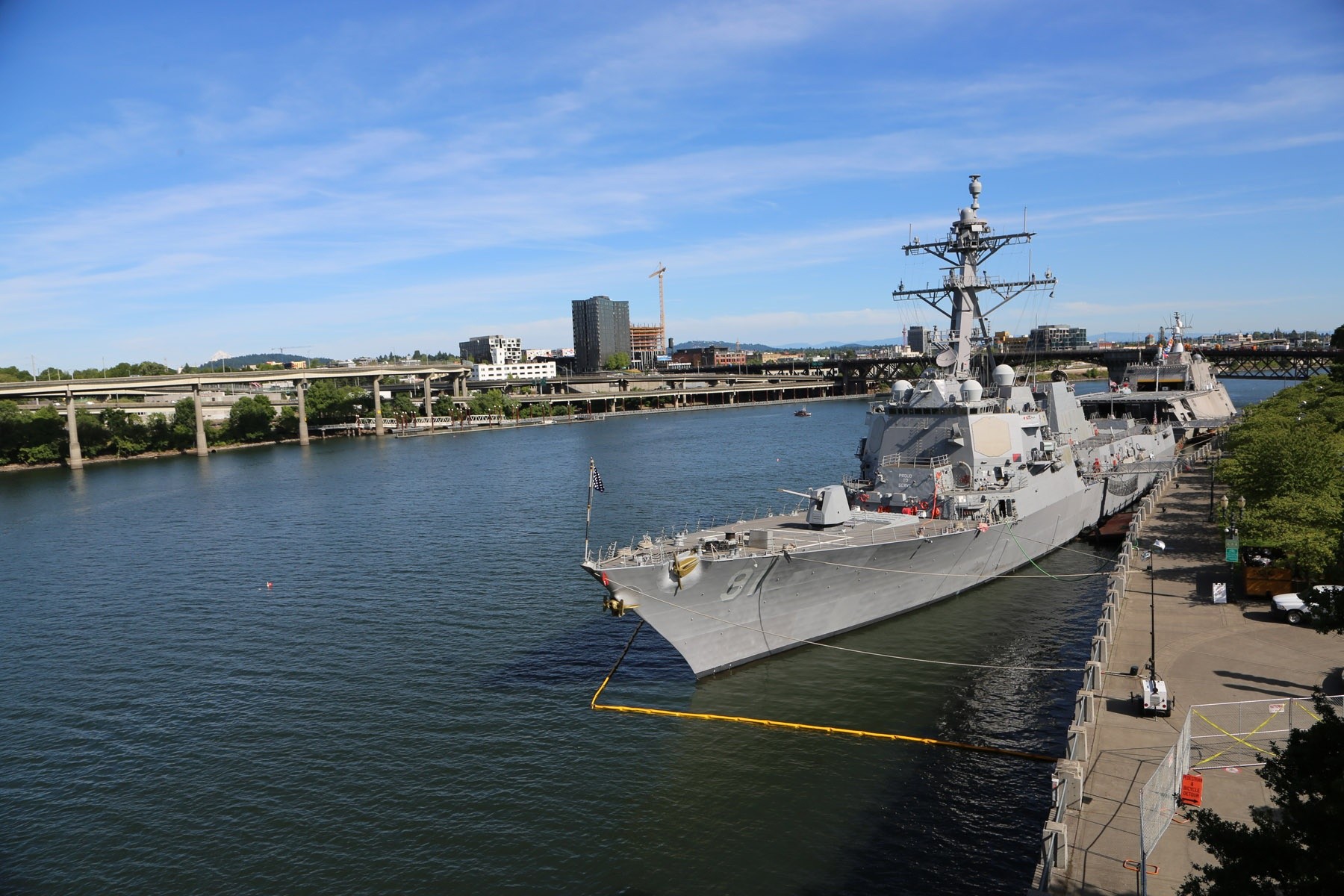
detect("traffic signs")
[1181,774,1203,806]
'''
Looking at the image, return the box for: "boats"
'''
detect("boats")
[578,175,1177,680]
[794,405,811,416]
[1074,311,1237,460]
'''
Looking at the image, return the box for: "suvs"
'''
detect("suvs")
[1271,585,1344,626]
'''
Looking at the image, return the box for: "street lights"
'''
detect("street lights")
[1219,494,1246,599]
[1204,447,1221,522]
[1215,425,1230,459]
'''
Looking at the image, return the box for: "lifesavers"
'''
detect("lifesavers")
[920,501,929,510]
[860,494,868,502]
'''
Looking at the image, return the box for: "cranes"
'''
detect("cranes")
[649,261,667,353]
